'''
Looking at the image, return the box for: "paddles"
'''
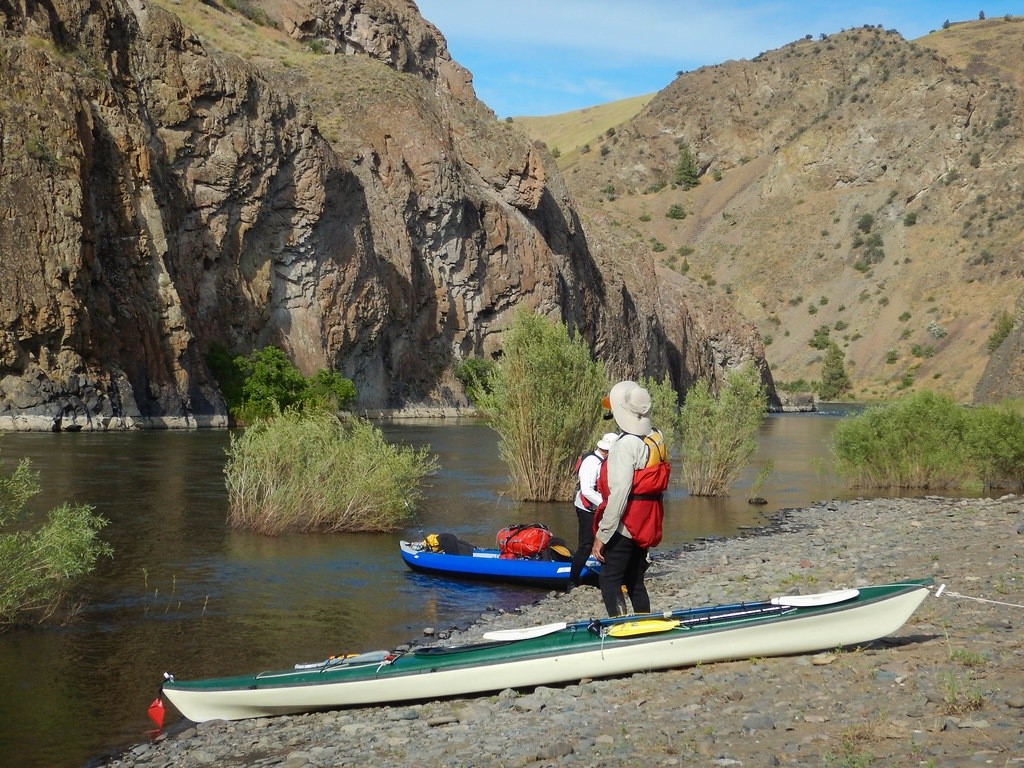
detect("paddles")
[550,545,628,592]
[482,587,861,643]
[605,605,792,638]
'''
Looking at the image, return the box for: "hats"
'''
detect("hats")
[597,433,619,451]
[609,380,653,436]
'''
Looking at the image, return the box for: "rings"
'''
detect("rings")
[595,553,598,556]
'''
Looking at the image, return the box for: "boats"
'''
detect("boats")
[400,537,657,583]
[158,578,935,726]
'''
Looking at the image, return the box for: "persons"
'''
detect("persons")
[593,381,671,617]
[567,433,619,592]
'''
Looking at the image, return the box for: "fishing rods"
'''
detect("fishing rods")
[380,449,433,552]
